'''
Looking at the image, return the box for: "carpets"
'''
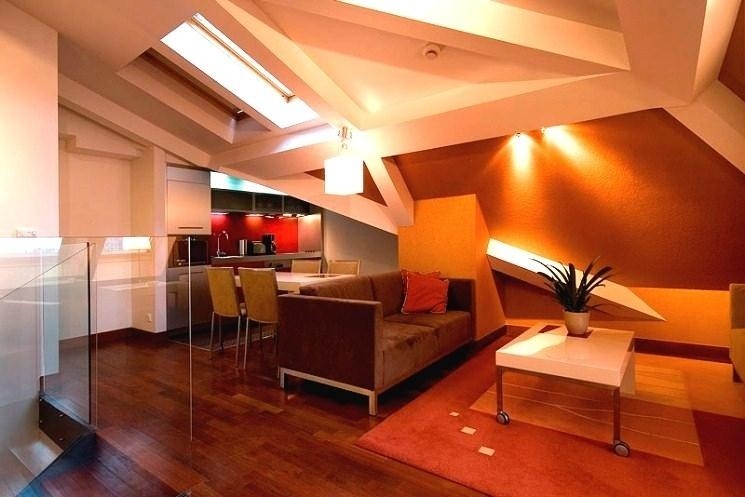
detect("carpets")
[351,331,745,496]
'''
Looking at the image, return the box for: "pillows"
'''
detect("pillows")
[398,269,442,298]
[400,272,451,317]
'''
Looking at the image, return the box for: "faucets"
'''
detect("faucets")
[216,228,228,255]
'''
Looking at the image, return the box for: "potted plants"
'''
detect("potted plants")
[527,252,619,340]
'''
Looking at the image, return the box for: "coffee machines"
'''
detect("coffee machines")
[261,232,276,254]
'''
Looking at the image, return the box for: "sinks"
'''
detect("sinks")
[214,255,244,258]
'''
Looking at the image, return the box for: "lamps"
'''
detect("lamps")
[323,122,365,196]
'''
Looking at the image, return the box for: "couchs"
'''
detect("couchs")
[720,277,745,386]
[275,269,480,418]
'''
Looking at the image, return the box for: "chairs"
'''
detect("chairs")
[236,265,283,373]
[290,256,324,274]
[327,258,362,276]
[202,263,264,361]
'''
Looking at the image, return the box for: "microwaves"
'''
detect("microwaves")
[171,237,209,265]
